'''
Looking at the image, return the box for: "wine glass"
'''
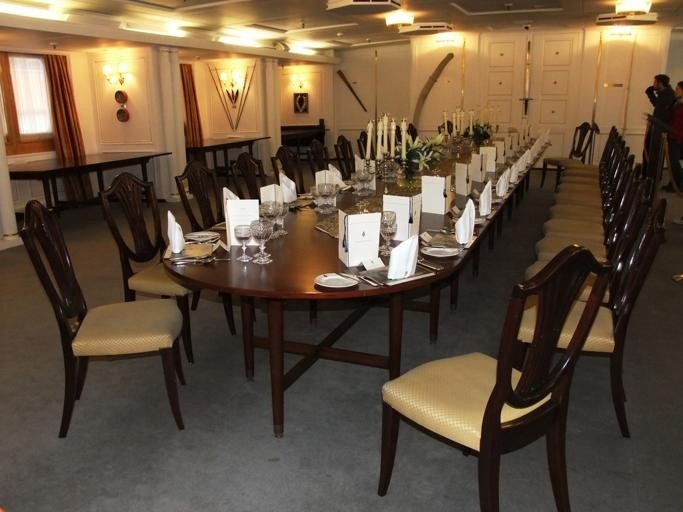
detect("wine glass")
[380,211,400,256]
[350,168,378,205]
[310,184,342,215]
[236,200,290,264]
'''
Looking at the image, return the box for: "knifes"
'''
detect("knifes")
[171,255,227,266]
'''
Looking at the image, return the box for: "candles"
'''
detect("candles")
[366,113,407,160]
[443,106,474,136]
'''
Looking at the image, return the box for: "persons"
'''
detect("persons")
[638,74,678,183]
[660,80,683,193]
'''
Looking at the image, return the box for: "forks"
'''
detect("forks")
[349,265,385,289]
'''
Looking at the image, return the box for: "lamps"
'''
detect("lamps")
[103,63,128,85]
[220,70,241,88]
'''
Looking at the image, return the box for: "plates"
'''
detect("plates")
[314,271,363,289]
[451,127,549,228]
[422,245,460,258]
[185,231,221,242]
